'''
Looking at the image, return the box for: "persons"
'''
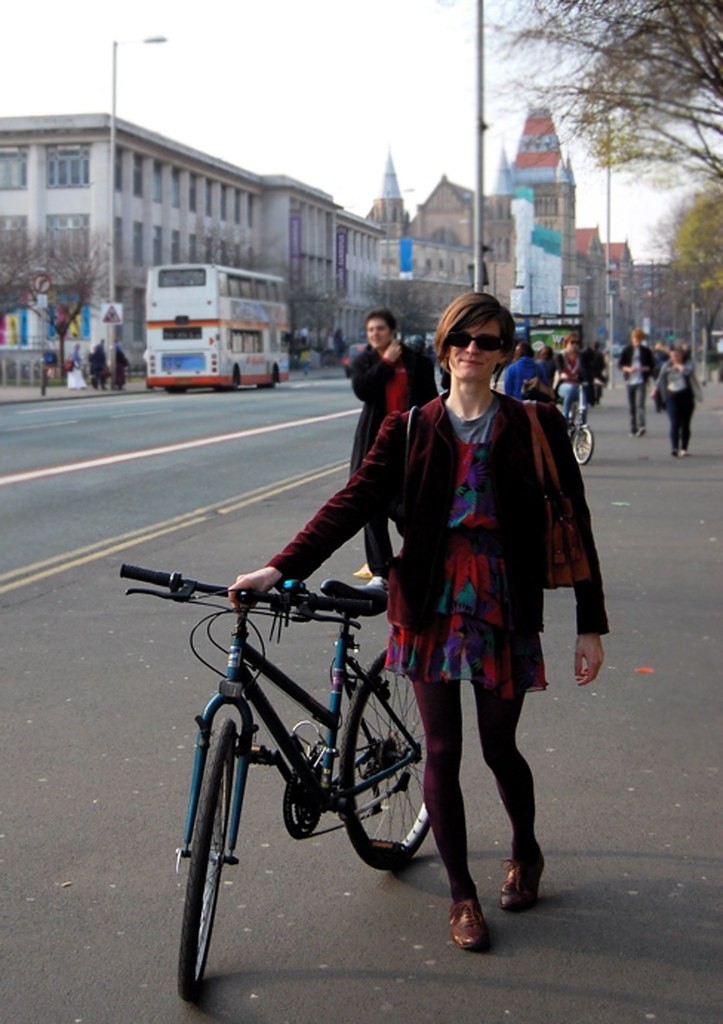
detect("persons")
[650,340,675,381]
[650,347,702,456]
[618,329,656,436]
[334,328,347,357]
[67,343,87,389]
[228,293,613,948]
[536,332,608,424]
[116,347,129,390]
[505,340,549,404]
[322,352,337,367]
[89,345,106,390]
[348,308,439,587]
[300,349,311,375]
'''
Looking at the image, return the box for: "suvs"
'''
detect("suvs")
[343,343,368,378]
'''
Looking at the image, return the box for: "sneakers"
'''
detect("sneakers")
[365,576,390,593]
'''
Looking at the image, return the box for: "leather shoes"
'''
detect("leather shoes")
[497,854,545,909]
[449,892,487,948]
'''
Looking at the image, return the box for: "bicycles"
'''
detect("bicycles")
[555,380,595,464]
[120,562,431,1002]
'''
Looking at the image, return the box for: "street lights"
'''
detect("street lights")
[571,116,614,389]
[109,38,167,389]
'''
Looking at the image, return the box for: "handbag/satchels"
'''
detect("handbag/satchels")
[522,398,591,589]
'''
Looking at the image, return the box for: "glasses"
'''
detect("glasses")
[445,329,504,352]
[570,340,580,344]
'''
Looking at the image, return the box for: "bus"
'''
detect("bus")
[145,263,291,393]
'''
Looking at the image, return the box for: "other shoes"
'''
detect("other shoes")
[671,449,679,458]
[636,427,645,437]
[679,449,690,457]
